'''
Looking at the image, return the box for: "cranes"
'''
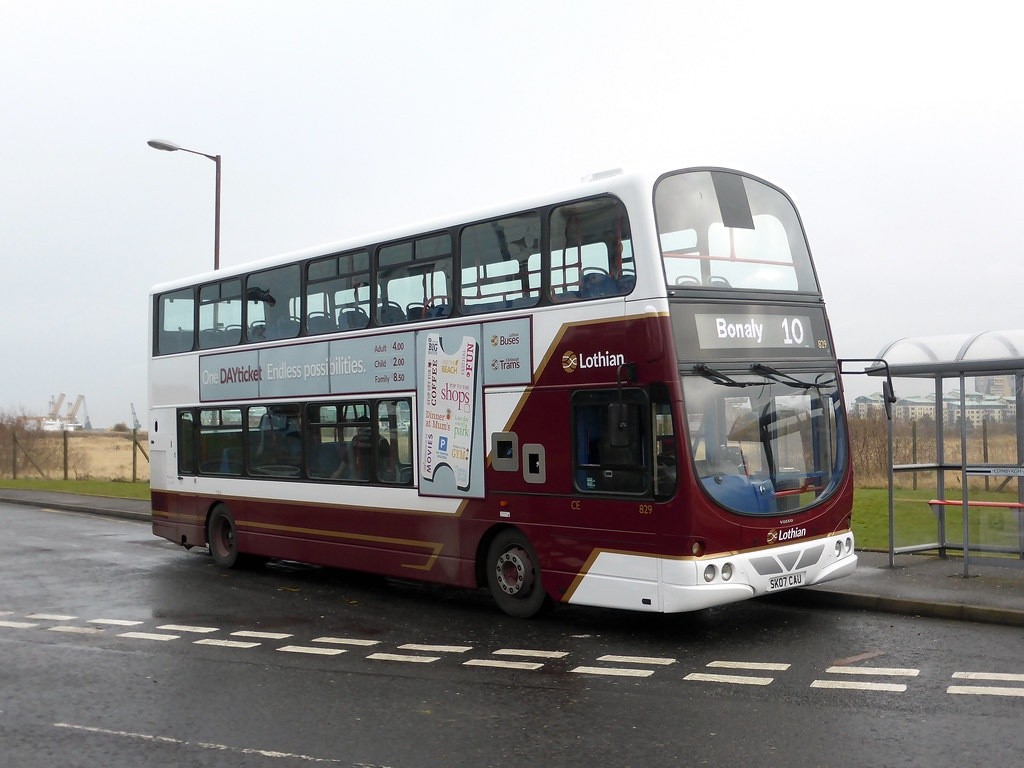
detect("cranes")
[131,402,142,430]
[66,395,84,418]
[49,394,66,417]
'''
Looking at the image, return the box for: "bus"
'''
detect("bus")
[147,162,897,622]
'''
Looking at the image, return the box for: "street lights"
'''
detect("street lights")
[147,139,221,425]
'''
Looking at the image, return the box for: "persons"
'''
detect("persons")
[351,416,393,480]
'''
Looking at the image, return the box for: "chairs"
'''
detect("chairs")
[614,269,636,294]
[376,301,405,325]
[338,307,368,330]
[406,302,431,321]
[200,329,224,348]
[224,324,250,345]
[306,311,337,334]
[675,276,700,287]
[707,275,732,289]
[579,267,616,299]
[277,316,301,338]
[250,320,277,342]
